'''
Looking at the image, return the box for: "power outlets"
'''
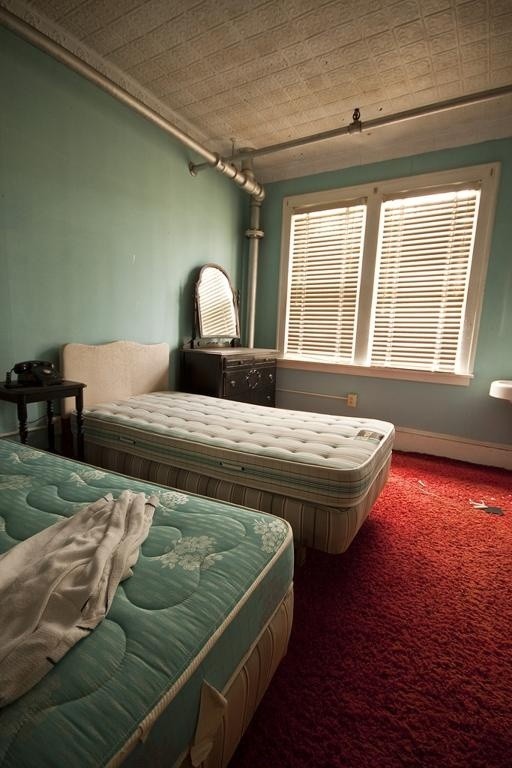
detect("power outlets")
[347,394,357,407]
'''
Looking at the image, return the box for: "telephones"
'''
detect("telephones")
[14,360,63,386]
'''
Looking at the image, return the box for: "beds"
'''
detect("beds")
[0,437,298,767]
[59,339,396,564]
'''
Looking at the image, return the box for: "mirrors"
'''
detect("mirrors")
[190,263,242,349]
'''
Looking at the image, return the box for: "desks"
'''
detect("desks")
[0,380,87,465]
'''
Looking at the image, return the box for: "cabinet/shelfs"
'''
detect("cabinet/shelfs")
[177,347,280,407]
